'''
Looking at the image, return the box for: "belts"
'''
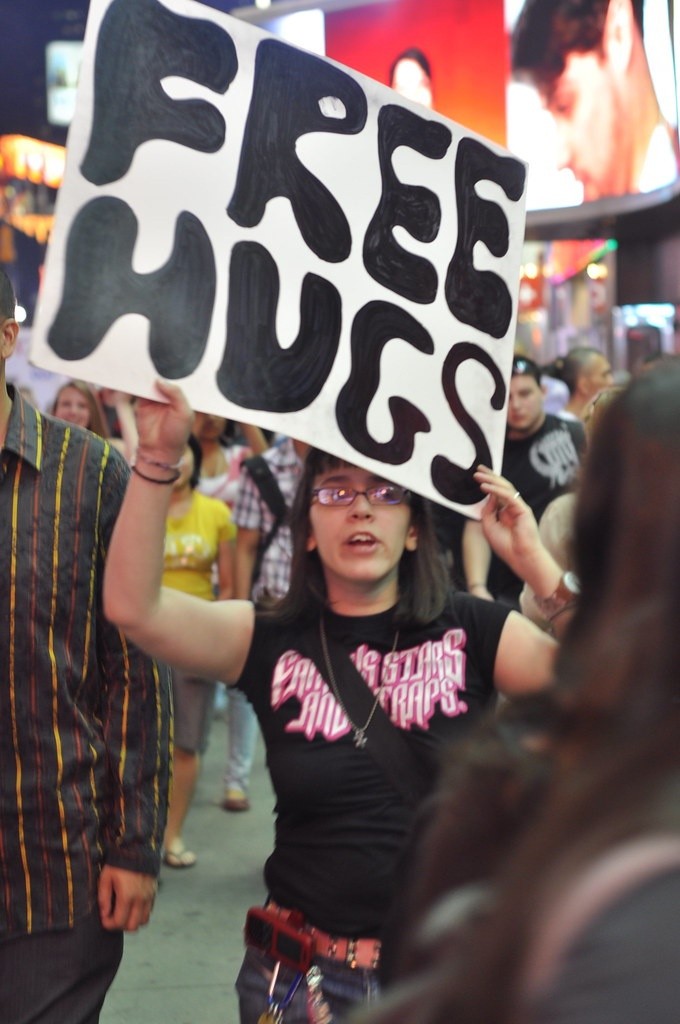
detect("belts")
[267,903,385,973]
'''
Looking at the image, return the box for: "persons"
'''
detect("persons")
[388,49,433,109]
[510,0,680,206]
[0,262,680,1024]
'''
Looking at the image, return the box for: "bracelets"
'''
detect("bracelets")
[137,449,180,471]
[542,602,577,631]
[131,466,181,484]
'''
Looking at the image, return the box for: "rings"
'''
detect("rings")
[513,491,520,500]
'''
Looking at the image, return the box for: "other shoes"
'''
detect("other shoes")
[223,796,248,810]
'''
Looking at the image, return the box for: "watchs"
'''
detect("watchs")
[538,572,579,622]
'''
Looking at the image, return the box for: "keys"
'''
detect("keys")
[257,1002,285,1024]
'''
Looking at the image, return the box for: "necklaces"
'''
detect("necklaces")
[319,605,400,749]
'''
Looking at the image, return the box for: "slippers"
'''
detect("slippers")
[168,848,197,867]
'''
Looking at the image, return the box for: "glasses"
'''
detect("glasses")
[510,357,539,377]
[309,485,408,505]
